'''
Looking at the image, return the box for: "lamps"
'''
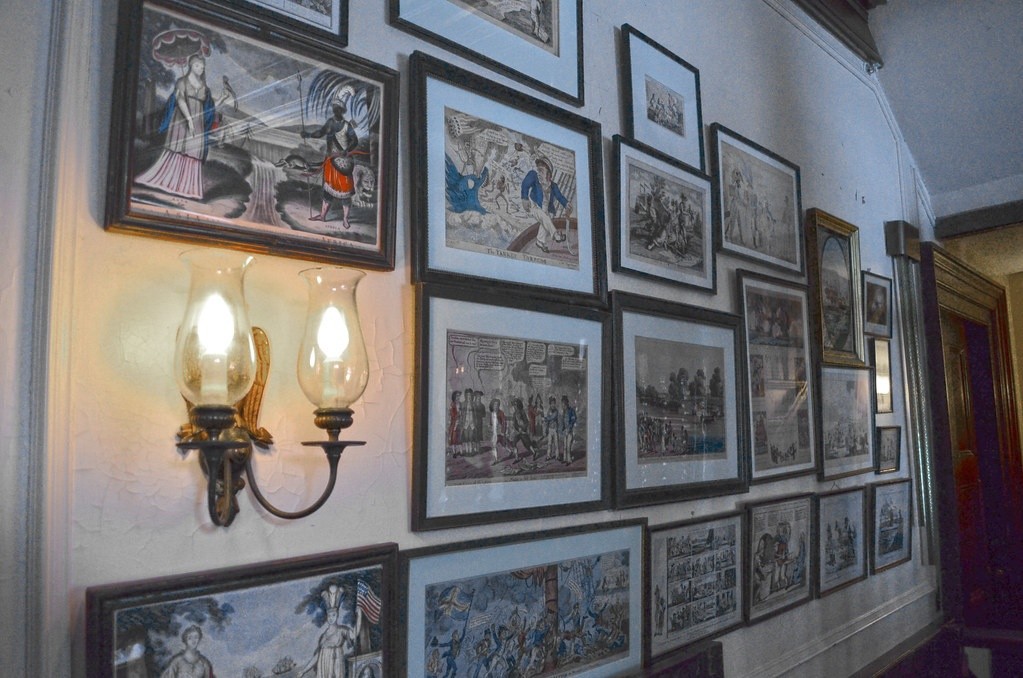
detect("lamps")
[180,248,366,525]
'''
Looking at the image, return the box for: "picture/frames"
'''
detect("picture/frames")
[82,0,919,678]
